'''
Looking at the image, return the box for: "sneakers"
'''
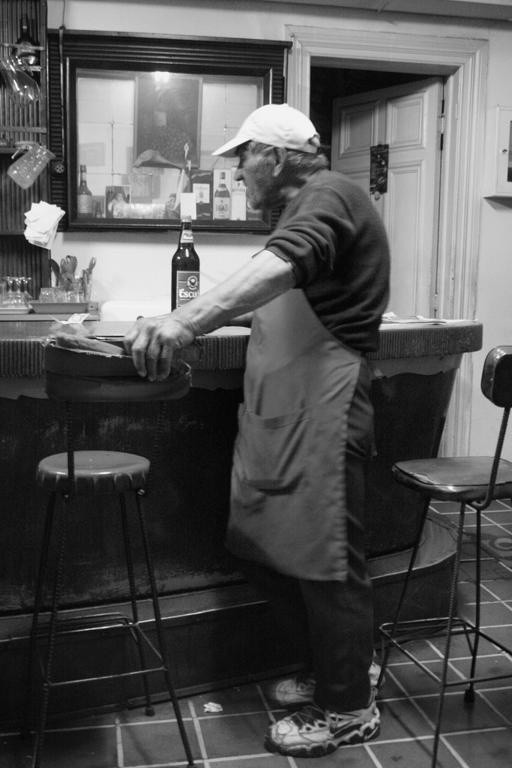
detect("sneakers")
[264,656,386,708]
[263,697,384,759]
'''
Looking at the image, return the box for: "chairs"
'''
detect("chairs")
[375,343,511,768]
[19,335,201,767]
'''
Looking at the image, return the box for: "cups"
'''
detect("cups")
[39,288,87,303]
[1,54,41,106]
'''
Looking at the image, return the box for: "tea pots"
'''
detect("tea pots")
[7,141,57,190]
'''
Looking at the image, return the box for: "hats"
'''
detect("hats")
[210,101,323,159]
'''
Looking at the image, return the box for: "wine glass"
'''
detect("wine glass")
[0,276,31,309]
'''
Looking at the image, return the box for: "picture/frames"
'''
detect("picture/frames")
[45,25,294,234]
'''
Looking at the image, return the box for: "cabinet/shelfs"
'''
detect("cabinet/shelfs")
[1,0,50,312]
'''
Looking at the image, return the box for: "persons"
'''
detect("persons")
[123,103,391,756]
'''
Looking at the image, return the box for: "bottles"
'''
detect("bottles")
[76,164,93,219]
[215,171,231,220]
[171,216,200,315]
